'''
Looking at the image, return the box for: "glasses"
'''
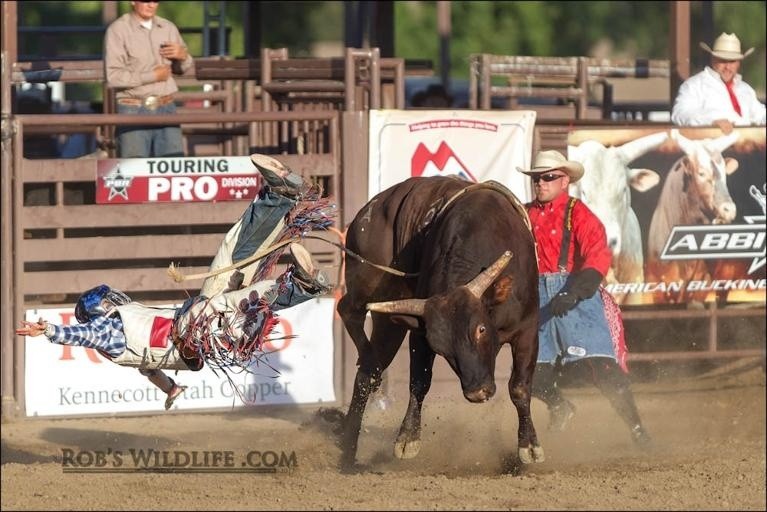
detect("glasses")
[534,173,565,183]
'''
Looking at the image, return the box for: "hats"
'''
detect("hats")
[699,33,755,61]
[517,150,584,184]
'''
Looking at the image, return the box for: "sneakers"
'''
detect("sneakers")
[251,154,303,195]
[630,424,646,445]
[547,401,575,432]
[290,243,328,289]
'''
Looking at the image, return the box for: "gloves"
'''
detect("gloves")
[548,268,603,318]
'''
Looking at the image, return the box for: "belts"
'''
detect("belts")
[118,94,175,110]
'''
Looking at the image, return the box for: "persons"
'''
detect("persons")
[15,155,331,411]
[102,1,196,158]
[666,27,765,133]
[514,151,658,471]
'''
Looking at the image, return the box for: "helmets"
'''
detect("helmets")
[75,284,132,324]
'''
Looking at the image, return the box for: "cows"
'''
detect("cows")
[647,127,745,300]
[333,174,546,470]
[567,127,668,306]
